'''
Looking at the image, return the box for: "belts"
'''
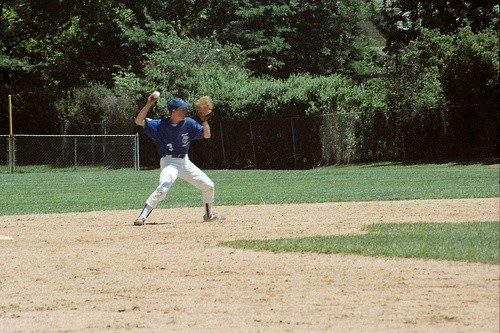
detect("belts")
[164,155,185,158]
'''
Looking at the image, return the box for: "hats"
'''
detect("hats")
[168,98,189,110]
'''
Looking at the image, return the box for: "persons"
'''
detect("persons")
[134,95,227,225]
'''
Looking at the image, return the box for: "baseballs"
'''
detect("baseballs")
[153,91,160,98]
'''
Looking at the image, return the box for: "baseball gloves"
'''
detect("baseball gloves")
[195,96,213,117]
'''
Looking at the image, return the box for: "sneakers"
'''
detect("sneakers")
[204,214,225,222]
[134,218,144,225]
